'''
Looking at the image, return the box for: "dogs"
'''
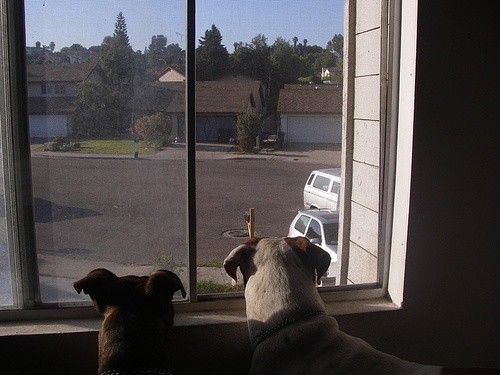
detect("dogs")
[222,235,445,374]
[72,268,187,375]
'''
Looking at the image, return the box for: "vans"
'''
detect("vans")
[303,167,340,209]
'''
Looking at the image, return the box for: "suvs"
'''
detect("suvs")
[287,208,339,278]
